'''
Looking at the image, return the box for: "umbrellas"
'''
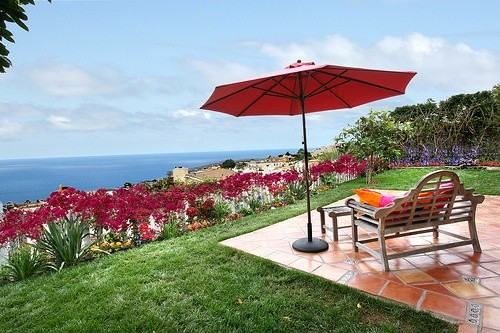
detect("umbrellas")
[199,60,418,252]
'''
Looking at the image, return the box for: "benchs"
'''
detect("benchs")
[345,170,486,272]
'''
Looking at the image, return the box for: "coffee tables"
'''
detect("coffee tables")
[317,206,352,241]
